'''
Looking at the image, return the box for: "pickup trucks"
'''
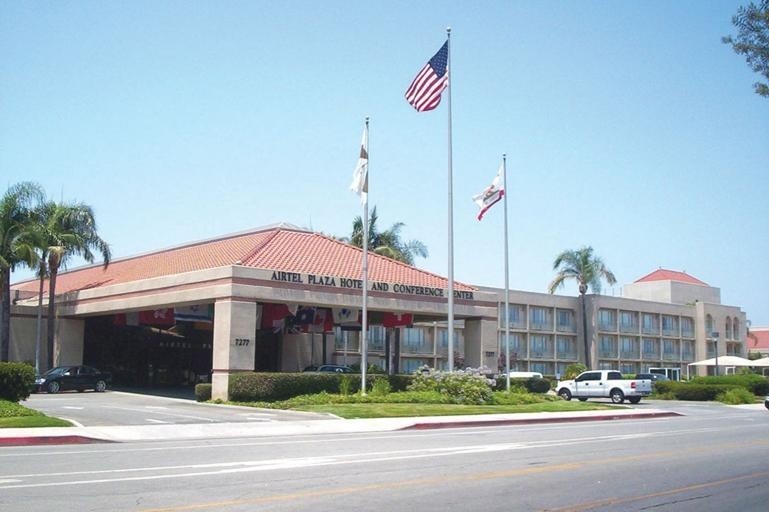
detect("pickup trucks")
[555,369,653,404]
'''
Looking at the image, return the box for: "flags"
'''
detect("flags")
[348,122,369,214]
[400,39,448,114]
[472,159,504,220]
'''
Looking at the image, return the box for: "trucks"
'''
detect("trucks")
[496,371,543,379]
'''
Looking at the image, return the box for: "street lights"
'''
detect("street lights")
[711,332,719,375]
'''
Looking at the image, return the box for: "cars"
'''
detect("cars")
[301,364,355,373]
[29,364,113,394]
